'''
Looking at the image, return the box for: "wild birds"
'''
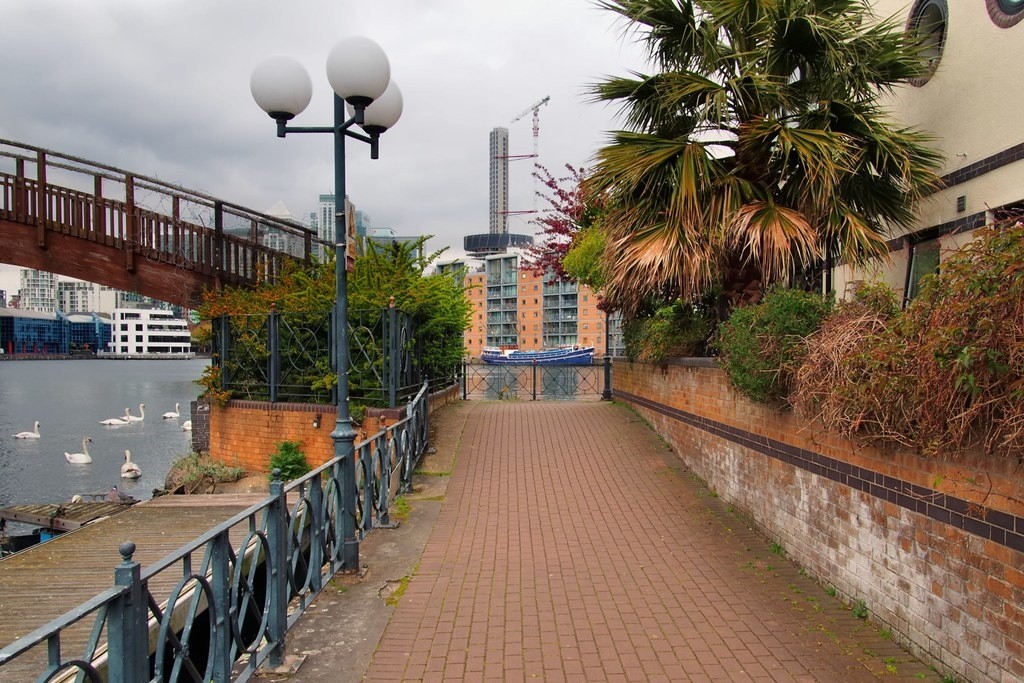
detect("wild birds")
[109,485,129,500]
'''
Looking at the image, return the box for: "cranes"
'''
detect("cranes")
[511,94,551,237]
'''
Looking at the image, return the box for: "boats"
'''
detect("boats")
[480,341,596,367]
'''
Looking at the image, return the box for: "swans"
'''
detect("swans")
[98,408,133,424]
[161,402,180,417]
[64,436,96,464]
[119,404,147,421]
[11,420,42,439]
[121,450,142,479]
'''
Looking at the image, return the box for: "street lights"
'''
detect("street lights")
[249,35,404,475]
